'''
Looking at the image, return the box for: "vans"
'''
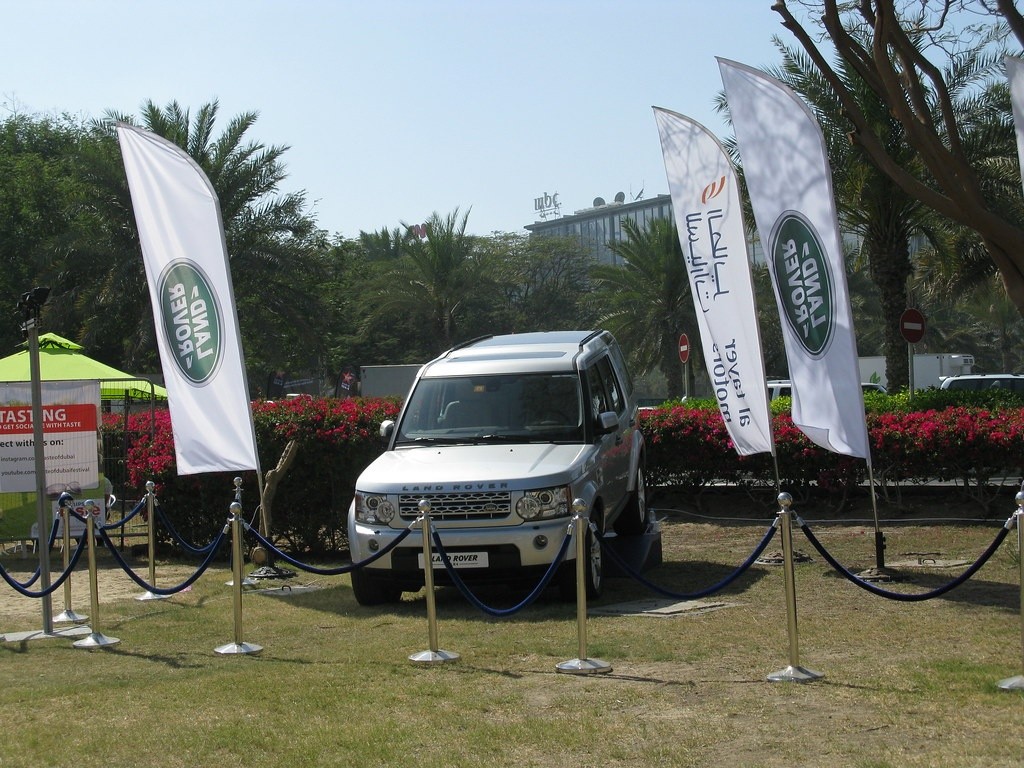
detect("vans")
[767,379,888,406]
[938,373,1024,399]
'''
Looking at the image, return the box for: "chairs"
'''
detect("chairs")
[437,383,494,429]
[546,379,578,423]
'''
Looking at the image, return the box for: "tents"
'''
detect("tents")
[0,332,168,400]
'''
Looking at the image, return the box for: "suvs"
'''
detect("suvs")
[347,327,649,606]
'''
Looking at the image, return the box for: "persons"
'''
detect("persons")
[72,476,112,549]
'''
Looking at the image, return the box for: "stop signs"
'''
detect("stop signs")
[899,308,925,344]
[678,334,688,364]
